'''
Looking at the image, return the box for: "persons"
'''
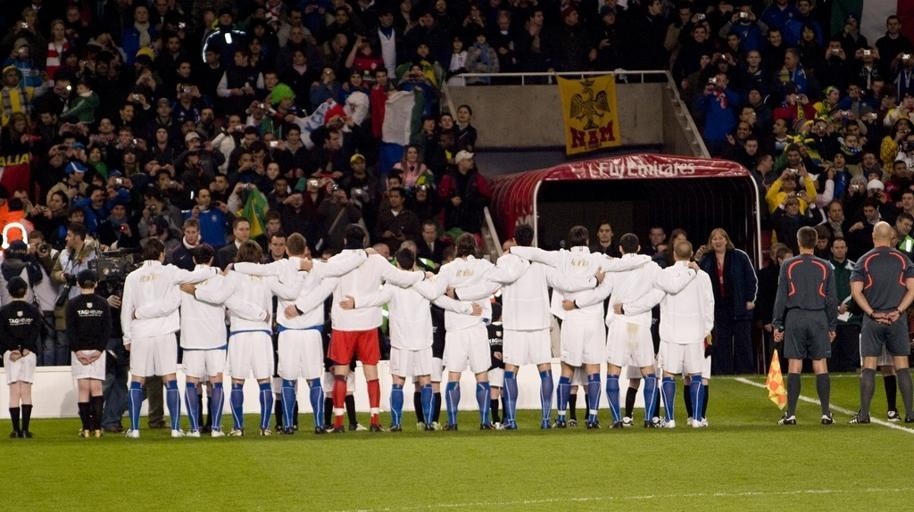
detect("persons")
[681,335,712,429]
[121,238,226,438]
[492,1,647,84]
[0,278,40,439]
[266,233,287,263]
[131,244,270,436]
[410,232,533,431]
[1,240,43,366]
[182,239,313,436]
[271,317,299,432]
[701,228,759,372]
[174,219,216,271]
[895,213,913,260]
[225,232,378,435]
[101,337,165,431]
[484,302,507,430]
[597,222,697,265]
[444,224,606,430]
[567,364,589,426]
[412,300,447,432]
[0,0,491,227]
[51,224,96,365]
[562,236,700,429]
[25,230,59,364]
[622,303,661,426]
[849,222,914,424]
[218,218,264,266]
[322,308,367,433]
[771,225,839,426]
[840,294,900,423]
[612,241,716,428]
[761,248,794,373]
[501,226,653,428]
[196,376,212,433]
[826,238,862,375]
[339,248,481,433]
[284,224,433,434]
[65,270,112,439]
[647,2,912,223]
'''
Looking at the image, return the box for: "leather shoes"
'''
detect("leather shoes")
[150,420,170,429]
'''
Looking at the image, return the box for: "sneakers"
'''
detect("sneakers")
[228,429,246,437]
[212,429,226,438]
[887,410,901,422]
[846,412,871,425]
[387,424,403,432]
[480,420,516,431]
[187,429,202,437]
[275,422,299,435]
[609,416,634,429]
[20,429,33,439]
[259,428,273,437]
[585,418,601,430]
[10,429,21,438]
[644,416,676,430]
[315,423,345,433]
[821,413,835,424]
[199,424,212,433]
[122,428,140,439]
[415,421,458,432]
[171,429,186,438]
[93,428,105,438]
[775,413,796,425]
[541,418,577,430]
[686,416,708,429]
[369,424,386,432]
[348,423,369,432]
[80,428,92,438]
[905,414,914,422]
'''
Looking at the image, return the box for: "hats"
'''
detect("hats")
[351,154,365,163]
[157,98,172,106]
[1,66,24,80]
[712,53,737,67]
[185,133,202,143]
[66,162,90,174]
[455,150,475,163]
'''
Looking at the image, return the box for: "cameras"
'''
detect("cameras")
[87,250,137,279]
[55,273,76,307]
[39,244,48,253]
[330,183,340,193]
